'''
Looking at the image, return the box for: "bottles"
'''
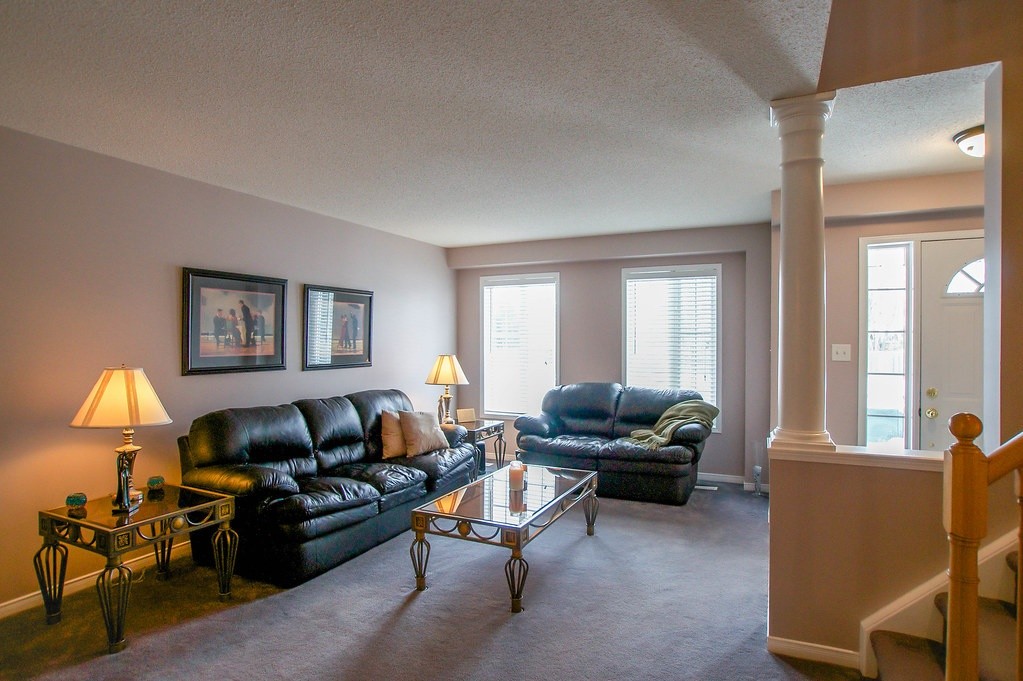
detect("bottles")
[509,461,524,491]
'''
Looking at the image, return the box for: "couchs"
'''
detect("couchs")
[175,387,479,589]
[515,380,712,508]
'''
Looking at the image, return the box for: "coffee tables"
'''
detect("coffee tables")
[406,460,600,615]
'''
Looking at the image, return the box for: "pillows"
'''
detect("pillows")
[398,409,449,461]
[376,407,404,463]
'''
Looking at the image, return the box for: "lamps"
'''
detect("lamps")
[68,362,174,503]
[424,353,470,426]
[435,487,468,514]
[952,121,985,158]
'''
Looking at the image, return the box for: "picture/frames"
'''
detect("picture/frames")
[179,267,290,377]
[301,282,375,373]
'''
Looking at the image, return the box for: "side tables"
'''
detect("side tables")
[28,480,242,654]
[437,409,508,470]
